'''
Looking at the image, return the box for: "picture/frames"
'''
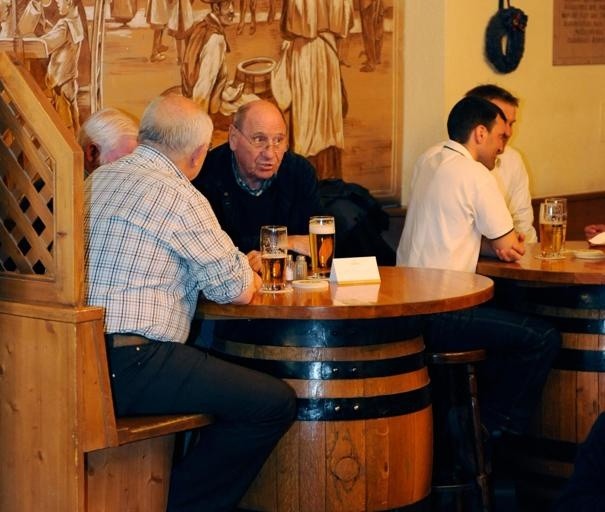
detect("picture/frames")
[0,0,405,219]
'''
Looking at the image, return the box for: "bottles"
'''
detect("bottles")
[293,255,308,280]
[534,197,567,261]
[285,254,294,281]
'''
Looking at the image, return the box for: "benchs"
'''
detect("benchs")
[0,301,211,512]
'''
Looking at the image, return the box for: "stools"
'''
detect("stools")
[430,348,489,511]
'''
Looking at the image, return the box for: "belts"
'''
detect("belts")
[113,334,149,349]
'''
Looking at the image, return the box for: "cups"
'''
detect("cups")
[307,214,337,283]
[259,225,287,291]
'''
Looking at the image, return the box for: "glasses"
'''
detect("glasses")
[234,124,286,150]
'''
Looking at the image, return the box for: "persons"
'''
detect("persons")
[583,224,605,240]
[412,82,541,248]
[392,93,566,470]
[85,93,303,512]
[188,97,329,253]
[0,102,142,274]
